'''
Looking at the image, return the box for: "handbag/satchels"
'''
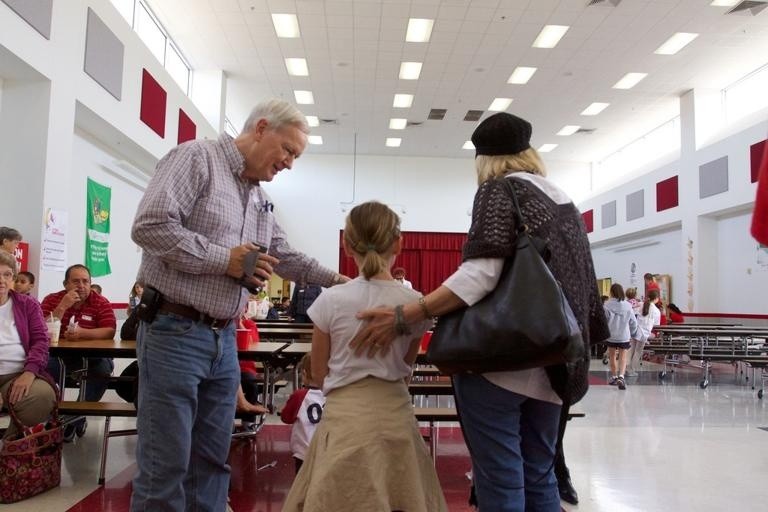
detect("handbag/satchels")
[424,230,587,376]
[0,422,65,504]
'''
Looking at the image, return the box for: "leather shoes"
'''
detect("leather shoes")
[558,475,578,505]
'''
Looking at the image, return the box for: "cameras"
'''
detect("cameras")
[234,241,268,295]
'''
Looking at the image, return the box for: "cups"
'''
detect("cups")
[46,317,64,346]
[420,330,433,352]
[233,329,253,350]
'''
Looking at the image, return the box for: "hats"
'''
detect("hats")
[471,112,532,159]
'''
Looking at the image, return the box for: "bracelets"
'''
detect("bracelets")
[394,305,412,337]
[418,297,432,321]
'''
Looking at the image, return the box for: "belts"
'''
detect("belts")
[154,298,236,329]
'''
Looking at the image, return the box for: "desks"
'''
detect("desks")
[646,323,767,403]
[41,314,585,491]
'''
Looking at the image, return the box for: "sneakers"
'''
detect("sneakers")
[63,425,76,445]
[76,420,88,437]
[590,353,610,365]
[608,367,640,390]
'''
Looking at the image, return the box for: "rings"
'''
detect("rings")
[374,342,383,348]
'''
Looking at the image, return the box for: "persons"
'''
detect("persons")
[591,273,684,390]
[280,202,450,512]
[276,296,290,314]
[281,351,326,476]
[393,267,412,289]
[0,226,117,442]
[234,290,274,429]
[129,98,352,512]
[554,403,579,505]
[116,281,144,410]
[348,112,610,512]
[290,284,322,323]
